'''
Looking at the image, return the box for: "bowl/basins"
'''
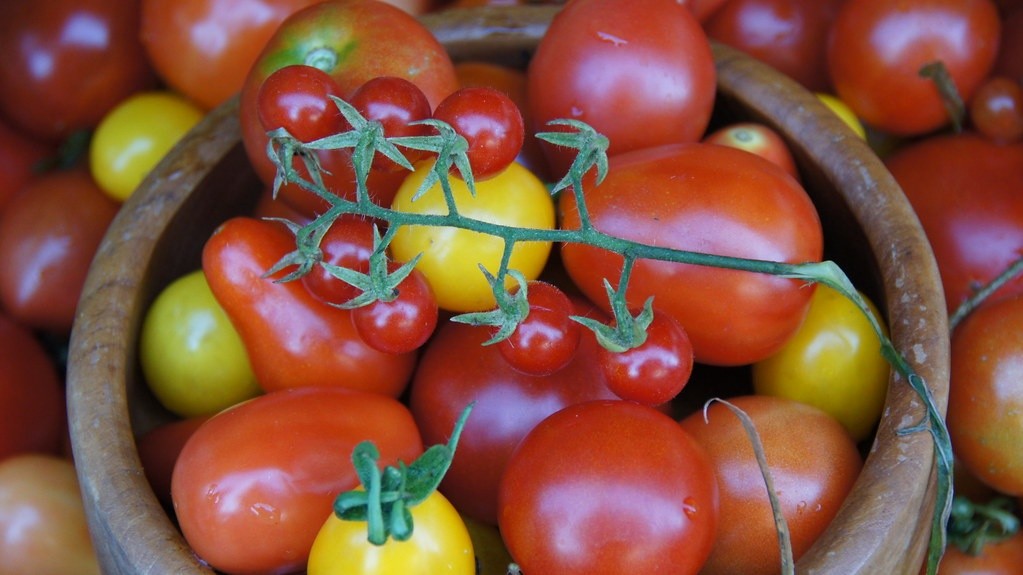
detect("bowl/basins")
[60,2,950,573]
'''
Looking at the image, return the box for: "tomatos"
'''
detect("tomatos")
[0,0,1023,575]
